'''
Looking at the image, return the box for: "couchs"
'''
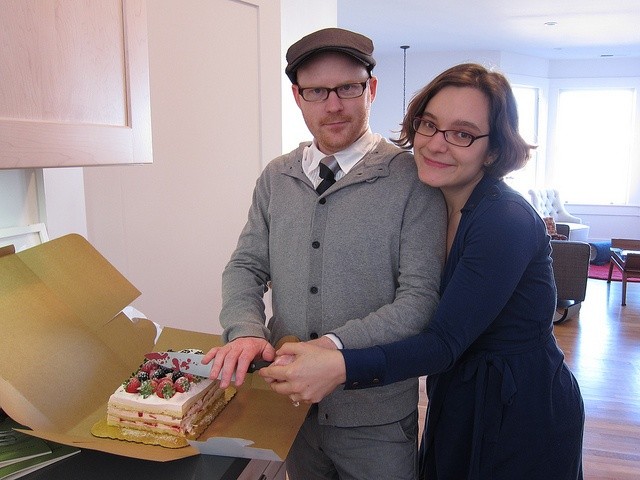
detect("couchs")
[541,224,591,326]
[528,187,589,242]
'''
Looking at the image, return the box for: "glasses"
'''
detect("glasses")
[295,76,371,102]
[412,114,492,148]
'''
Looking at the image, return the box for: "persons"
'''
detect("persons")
[258,64,586,480]
[201,27,449,480]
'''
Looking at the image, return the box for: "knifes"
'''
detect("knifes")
[144,352,273,383]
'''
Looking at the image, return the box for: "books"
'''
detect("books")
[0,441,82,480]
[0,422,53,468]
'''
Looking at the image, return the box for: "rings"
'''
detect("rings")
[293,400,303,408]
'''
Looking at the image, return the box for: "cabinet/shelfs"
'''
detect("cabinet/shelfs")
[0,0,157,170]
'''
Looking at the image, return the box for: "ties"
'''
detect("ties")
[314,154,340,196]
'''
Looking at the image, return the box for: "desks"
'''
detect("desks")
[605,238,640,307]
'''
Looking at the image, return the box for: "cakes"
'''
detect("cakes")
[106,349,226,436]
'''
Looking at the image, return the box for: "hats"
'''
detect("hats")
[285,27,377,84]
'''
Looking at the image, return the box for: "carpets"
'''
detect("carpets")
[588,262,640,282]
